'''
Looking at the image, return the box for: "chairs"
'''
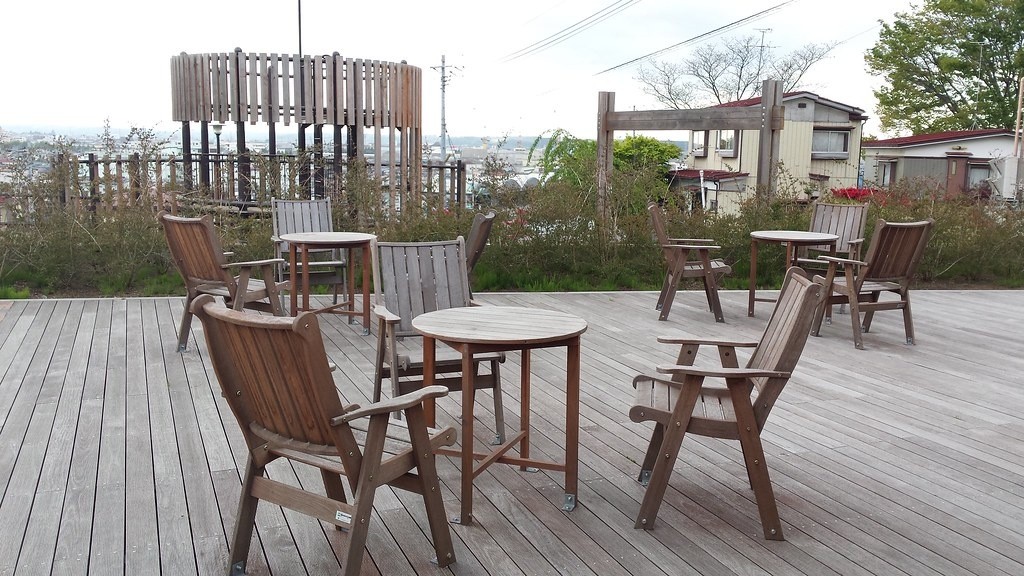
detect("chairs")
[647,202,731,323]
[189,293,458,576]
[157,210,285,354]
[271,197,350,317]
[781,202,870,282]
[371,212,509,445]
[810,217,937,350]
[628,266,825,541]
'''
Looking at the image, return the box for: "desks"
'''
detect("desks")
[411,307,589,526]
[748,231,840,322]
[279,233,378,337]
